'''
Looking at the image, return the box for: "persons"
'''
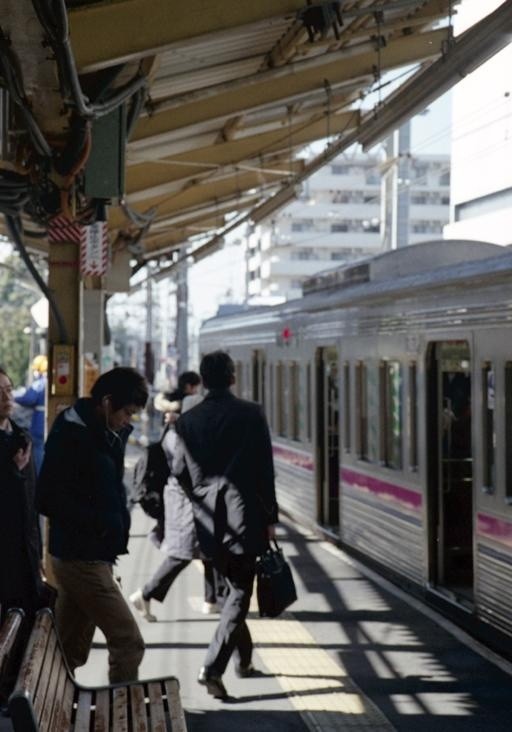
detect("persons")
[175,351,281,701]
[130,372,216,624]
[2,355,49,606]
[34,366,148,685]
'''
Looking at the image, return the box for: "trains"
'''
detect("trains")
[193,238,512,649]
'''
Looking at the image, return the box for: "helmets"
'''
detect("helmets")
[31,355,47,370]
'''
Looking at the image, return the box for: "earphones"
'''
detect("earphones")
[104,398,109,405]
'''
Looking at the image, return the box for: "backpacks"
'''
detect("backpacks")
[131,424,176,525]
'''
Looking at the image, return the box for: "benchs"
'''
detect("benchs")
[0,608,25,717]
[10,608,186,732]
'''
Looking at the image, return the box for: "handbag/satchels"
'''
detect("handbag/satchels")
[255,532,296,620]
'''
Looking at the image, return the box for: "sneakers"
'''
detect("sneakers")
[235,664,263,679]
[202,601,221,615]
[129,593,159,624]
[198,667,227,701]
